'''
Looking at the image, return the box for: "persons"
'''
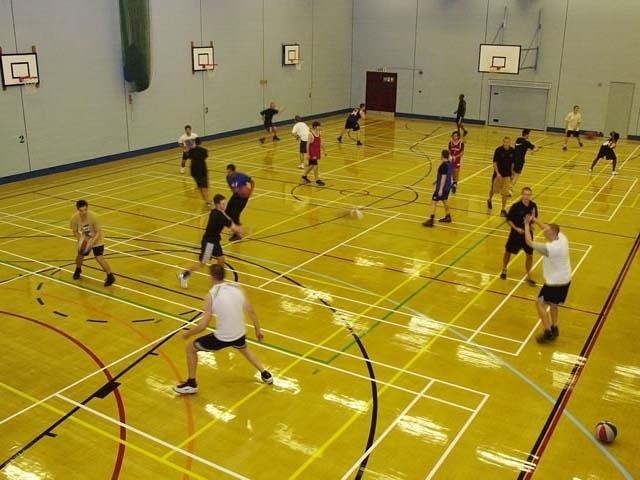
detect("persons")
[523,209,572,344]
[447,132,465,194]
[301,121,326,185]
[563,104,585,151]
[510,130,542,187]
[172,262,274,394]
[452,93,469,137]
[225,164,256,241]
[290,114,309,169]
[336,102,368,145]
[487,137,514,217]
[258,101,282,145]
[587,131,620,175]
[421,149,454,227]
[185,137,212,208]
[500,186,539,286]
[177,126,199,173]
[70,198,115,287]
[179,193,244,290]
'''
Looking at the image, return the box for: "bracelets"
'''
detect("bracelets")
[498,175,501,177]
[511,173,514,175]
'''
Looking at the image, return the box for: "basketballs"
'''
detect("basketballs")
[78,238,90,254]
[239,186,250,198]
[596,421,617,442]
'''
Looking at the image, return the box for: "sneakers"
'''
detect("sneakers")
[508,186,512,197]
[551,324,559,337]
[423,219,433,226]
[180,167,185,174]
[537,330,554,343]
[301,175,310,183]
[177,272,188,289]
[440,216,451,222]
[316,180,324,185]
[296,165,304,169]
[229,234,243,241]
[261,372,274,385]
[487,200,492,209]
[273,135,280,141]
[73,268,82,279]
[173,383,199,394]
[500,210,507,217]
[104,276,115,286]
[452,184,456,193]
[501,272,506,278]
